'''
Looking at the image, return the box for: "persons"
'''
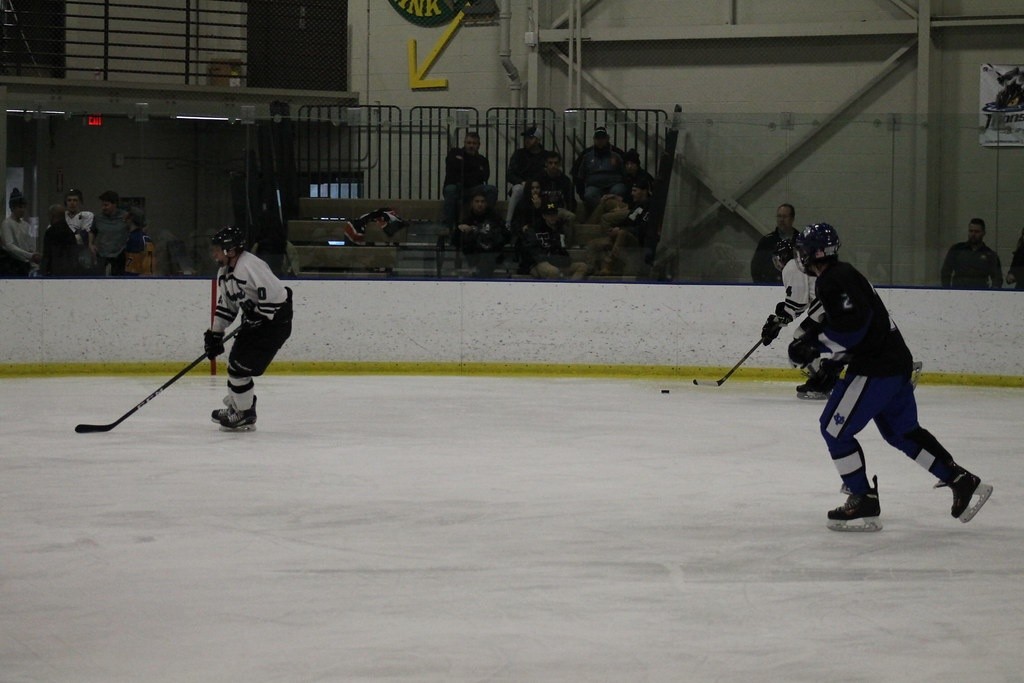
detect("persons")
[533,151,577,248]
[1006,225,1024,289]
[570,126,628,210]
[0,188,153,276]
[789,222,981,521]
[441,132,490,207]
[606,148,655,248]
[750,203,801,283]
[761,238,914,393]
[941,218,1003,289]
[204,225,293,428]
[505,126,550,231]
[510,178,563,279]
[452,192,513,278]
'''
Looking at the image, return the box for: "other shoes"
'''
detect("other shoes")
[795,378,831,401]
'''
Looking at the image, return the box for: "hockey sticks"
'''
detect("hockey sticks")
[692,317,787,387]
[807,364,831,399]
[75,323,242,434]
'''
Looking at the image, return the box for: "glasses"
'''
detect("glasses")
[525,135,536,138]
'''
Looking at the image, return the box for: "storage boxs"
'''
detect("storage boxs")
[206,59,242,88]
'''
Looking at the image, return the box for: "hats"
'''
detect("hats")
[63,188,84,205]
[519,126,543,141]
[632,179,651,197]
[9,188,27,208]
[593,127,610,138]
[624,148,640,163]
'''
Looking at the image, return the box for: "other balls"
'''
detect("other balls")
[661,389,669,393]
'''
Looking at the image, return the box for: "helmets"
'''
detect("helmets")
[797,222,846,274]
[775,231,802,257]
[210,225,246,250]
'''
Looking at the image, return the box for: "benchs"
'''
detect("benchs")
[287,197,603,277]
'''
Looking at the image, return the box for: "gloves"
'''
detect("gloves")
[202,329,226,360]
[759,314,783,346]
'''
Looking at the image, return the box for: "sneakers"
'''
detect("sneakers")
[210,394,258,433]
[824,483,884,533]
[937,466,995,524]
